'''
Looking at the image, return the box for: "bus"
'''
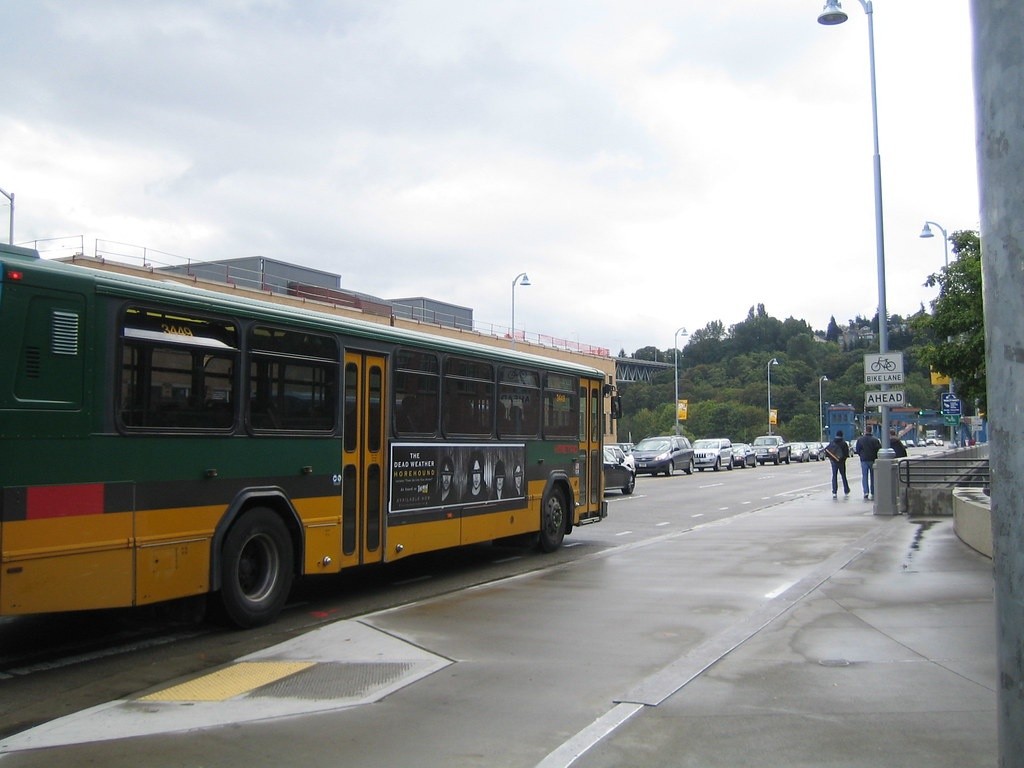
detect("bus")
[0,243,623,631]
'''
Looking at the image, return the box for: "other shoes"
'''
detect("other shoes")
[844,494,850,499]
[869,495,874,500]
[832,494,837,499]
[864,494,868,498]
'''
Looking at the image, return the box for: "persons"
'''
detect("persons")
[508,464,524,499]
[459,450,489,503]
[825,430,850,498]
[438,457,459,505]
[964,436,969,446]
[488,460,511,501]
[391,394,540,435]
[889,429,907,458]
[970,436,975,445]
[856,425,882,500]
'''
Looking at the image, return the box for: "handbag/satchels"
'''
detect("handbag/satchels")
[825,442,845,462]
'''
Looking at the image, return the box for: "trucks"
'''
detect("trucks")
[925,430,937,445]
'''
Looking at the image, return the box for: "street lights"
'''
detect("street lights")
[675,327,688,437]
[818,0,896,521]
[511,272,530,352]
[919,222,954,445]
[818,376,828,441]
[767,358,779,436]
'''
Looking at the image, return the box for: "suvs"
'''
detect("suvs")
[631,434,695,476]
[604,446,635,472]
[692,438,734,471]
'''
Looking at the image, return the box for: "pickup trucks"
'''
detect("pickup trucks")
[750,435,791,465]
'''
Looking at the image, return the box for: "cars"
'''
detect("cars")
[917,440,927,447]
[851,440,858,454]
[786,442,811,462]
[822,443,830,458]
[844,441,854,457]
[732,443,757,468]
[900,440,908,448]
[936,439,944,446]
[603,451,636,495]
[805,442,826,461]
[906,440,915,447]
